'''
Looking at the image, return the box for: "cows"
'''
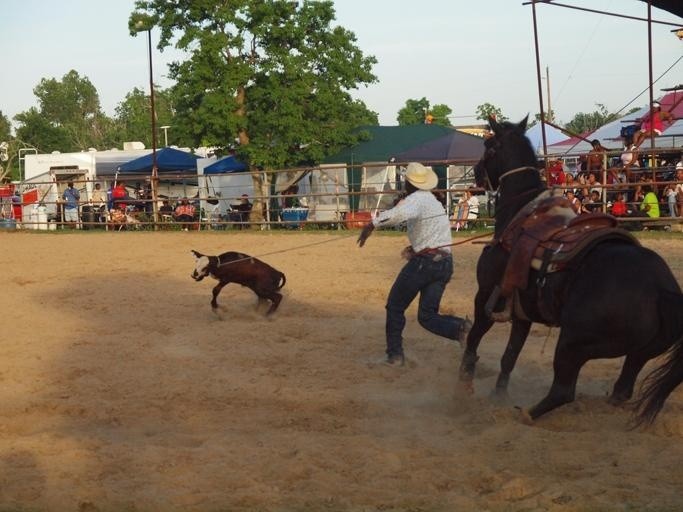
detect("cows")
[189,248,287,322]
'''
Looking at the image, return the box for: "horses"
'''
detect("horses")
[462,113,683,436]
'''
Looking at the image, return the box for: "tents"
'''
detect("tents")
[203,122,489,216]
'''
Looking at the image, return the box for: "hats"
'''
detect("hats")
[653,102,661,108]
[403,162,439,192]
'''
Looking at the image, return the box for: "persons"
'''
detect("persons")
[61,181,80,230]
[539,139,683,232]
[355,163,479,369]
[631,100,672,150]
[283,192,300,209]
[236,194,252,230]
[91,180,195,231]
[456,186,479,229]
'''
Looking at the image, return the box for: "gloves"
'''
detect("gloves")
[357,222,375,248]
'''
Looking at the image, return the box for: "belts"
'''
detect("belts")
[420,247,448,257]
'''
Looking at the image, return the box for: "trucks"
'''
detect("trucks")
[0,184,21,223]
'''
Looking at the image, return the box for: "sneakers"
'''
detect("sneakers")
[382,351,405,368]
[458,319,473,349]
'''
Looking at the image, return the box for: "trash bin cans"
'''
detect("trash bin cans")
[281,208,308,229]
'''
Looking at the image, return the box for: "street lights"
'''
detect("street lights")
[159,125,171,149]
[318,172,341,231]
[540,75,552,123]
